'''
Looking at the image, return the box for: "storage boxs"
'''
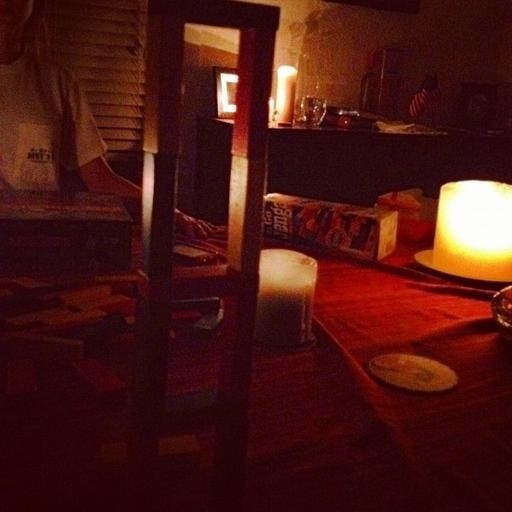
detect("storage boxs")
[2,188,134,273]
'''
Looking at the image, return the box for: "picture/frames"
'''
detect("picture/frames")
[212,65,240,119]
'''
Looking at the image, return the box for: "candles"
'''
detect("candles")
[432,180,512,282]
[276,64,298,122]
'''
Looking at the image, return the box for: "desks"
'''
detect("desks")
[193,111,465,230]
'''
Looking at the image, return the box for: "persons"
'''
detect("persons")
[1,1,217,242]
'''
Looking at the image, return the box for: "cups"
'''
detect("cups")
[294,94,329,127]
